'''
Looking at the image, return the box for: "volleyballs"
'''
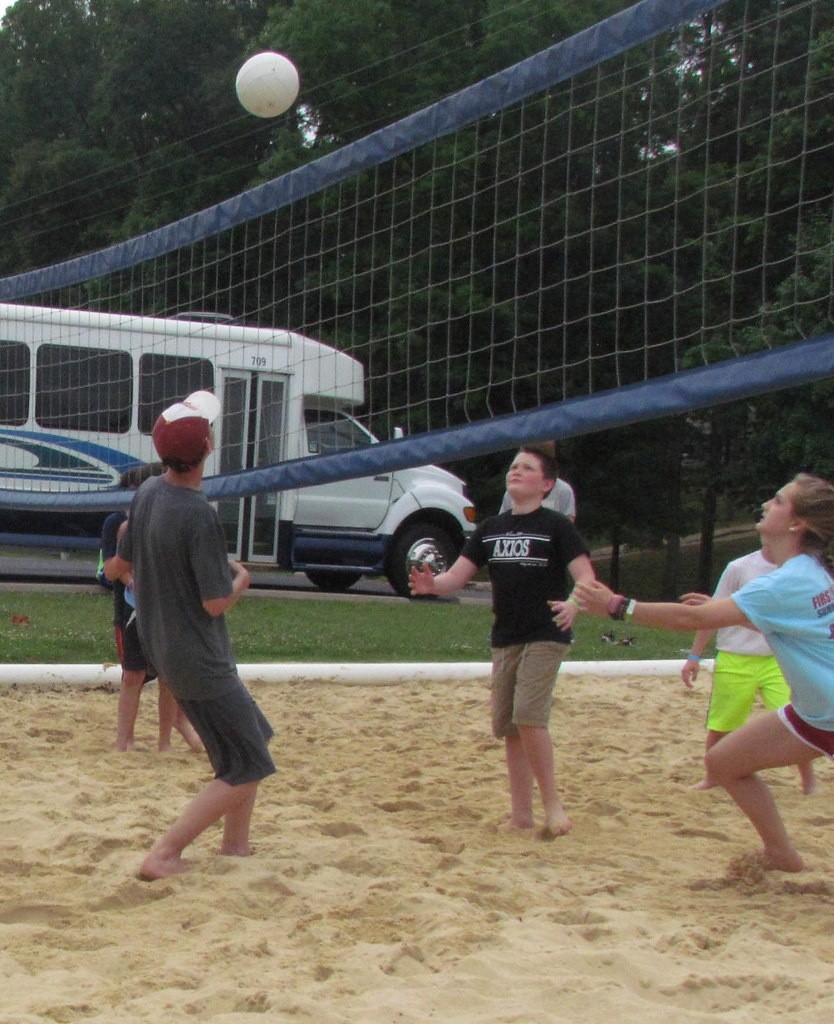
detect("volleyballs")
[235,52,301,119]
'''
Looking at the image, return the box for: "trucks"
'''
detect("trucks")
[0,302,480,601]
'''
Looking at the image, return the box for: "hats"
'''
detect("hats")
[153,390,222,464]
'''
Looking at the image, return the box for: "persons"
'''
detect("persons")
[681,509,821,796]
[117,390,274,881]
[575,471,834,870]
[99,465,207,754]
[407,440,596,838]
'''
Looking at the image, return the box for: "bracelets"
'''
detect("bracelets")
[567,597,581,607]
[129,579,134,588]
[687,655,700,660]
[607,594,637,623]
